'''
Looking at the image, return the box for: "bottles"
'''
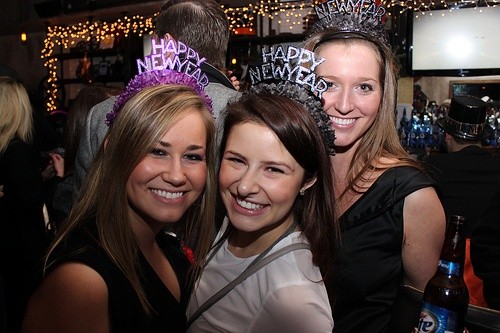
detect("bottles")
[416,214,470,333]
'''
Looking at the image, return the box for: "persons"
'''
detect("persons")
[185,45,334,333]
[299,0,447,333]
[72,0,247,199]
[0,76,121,333]
[22,38,217,333]
[424,96,500,313]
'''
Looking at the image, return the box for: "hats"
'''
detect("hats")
[436,95,495,142]
